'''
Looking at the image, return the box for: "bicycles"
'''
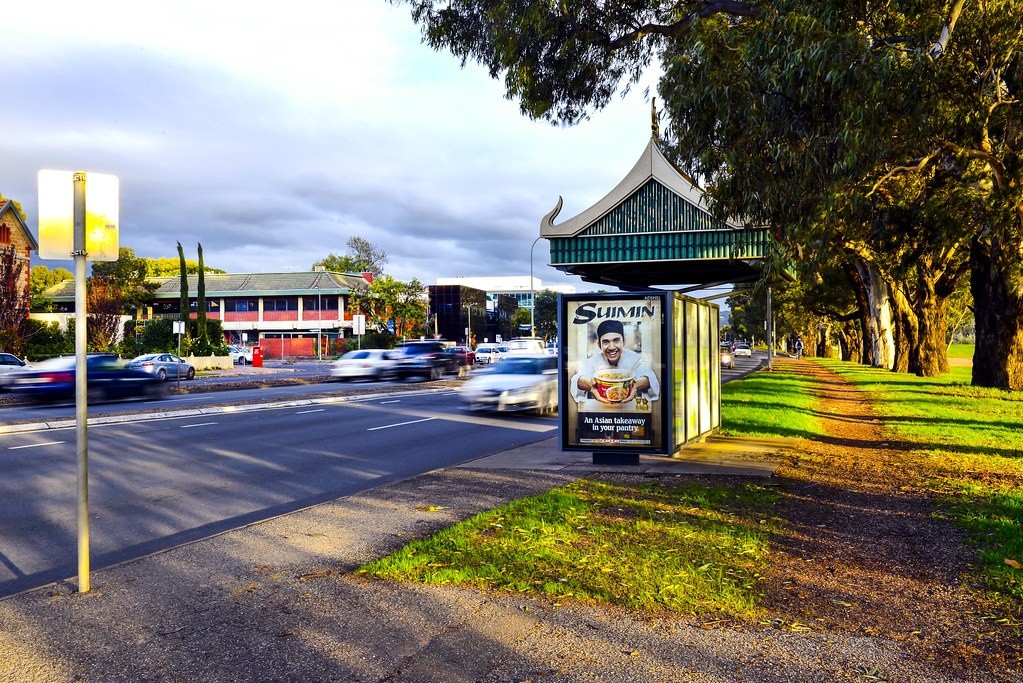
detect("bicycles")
[797,346,802,360]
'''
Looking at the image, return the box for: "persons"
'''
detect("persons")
[471,334,476,351]
[569,320,660,404]
[795,338,804,359]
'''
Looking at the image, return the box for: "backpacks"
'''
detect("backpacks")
[798,341,801,346]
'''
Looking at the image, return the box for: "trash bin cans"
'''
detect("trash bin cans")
[252,346,264,367]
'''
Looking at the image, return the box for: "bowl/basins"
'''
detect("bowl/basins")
[594,369,635,402]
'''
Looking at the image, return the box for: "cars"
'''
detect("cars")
[227,345,253,365]
[721,341,744,370]
[329,349,405,382]
[4,355,164,405]
[124,353,196,380]
[0,353,32,386]
[396,352,465,382]
[458,354,559,415]
[394,339,558,358]
[474,348,503,365]
[445,347,475,365]
[734,345,751,358]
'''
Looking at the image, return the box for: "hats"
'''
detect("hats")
[798,338,801,340]
[597,320,623,342]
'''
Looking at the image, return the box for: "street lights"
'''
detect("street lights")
[462,302,478,347]
[315,285,322,362]
